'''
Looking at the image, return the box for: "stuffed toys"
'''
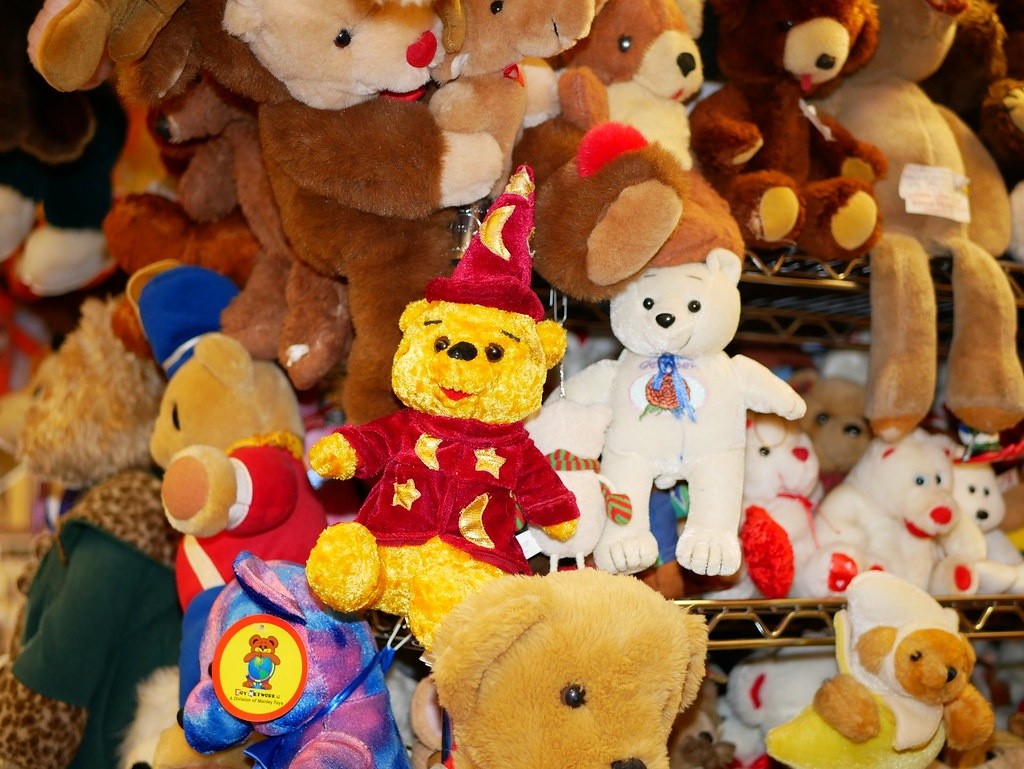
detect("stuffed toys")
[1,0,1023,769]
